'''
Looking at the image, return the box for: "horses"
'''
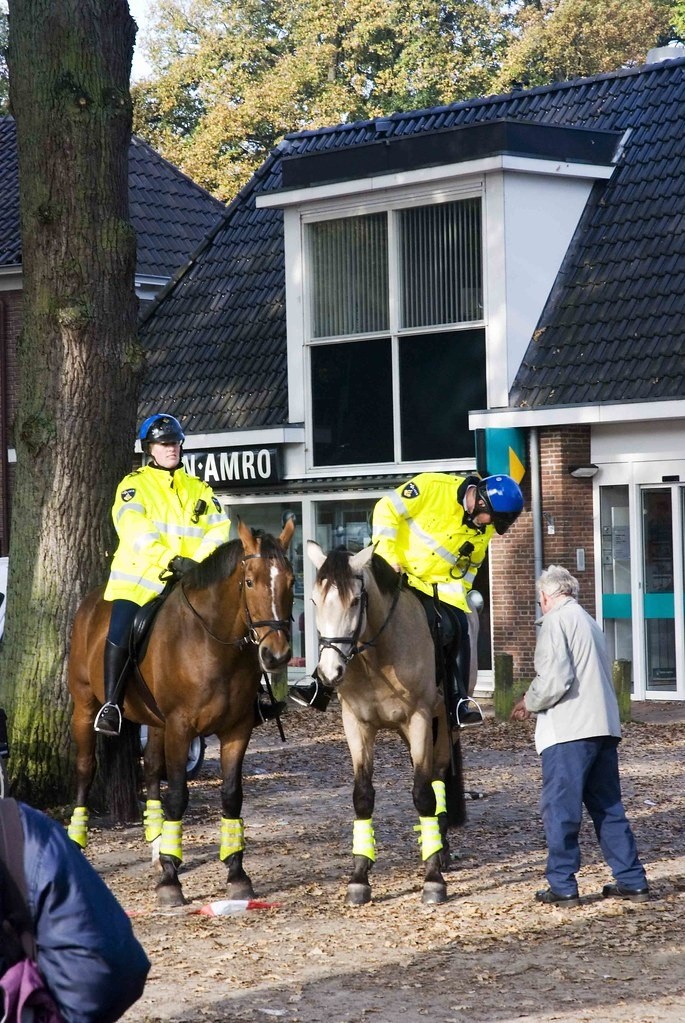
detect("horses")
[68,512,479,907]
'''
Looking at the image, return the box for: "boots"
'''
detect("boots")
[290,675,336,711]
[252,700,286,729]
[97,638,133,738]
[448,637,482,727]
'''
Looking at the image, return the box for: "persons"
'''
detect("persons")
[290,471,524,728]
[0,797,152,1023]
[509,566,651,908]
[94,412,287,731]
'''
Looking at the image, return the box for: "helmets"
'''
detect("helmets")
[140,413,185,451]
[477,475,524,535]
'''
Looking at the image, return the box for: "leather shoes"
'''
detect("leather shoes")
[536,888,580,907]
[603,881,650,902]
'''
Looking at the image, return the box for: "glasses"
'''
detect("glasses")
[537,599,543,607]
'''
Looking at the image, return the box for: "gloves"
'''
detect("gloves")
[168,555,201,578]
